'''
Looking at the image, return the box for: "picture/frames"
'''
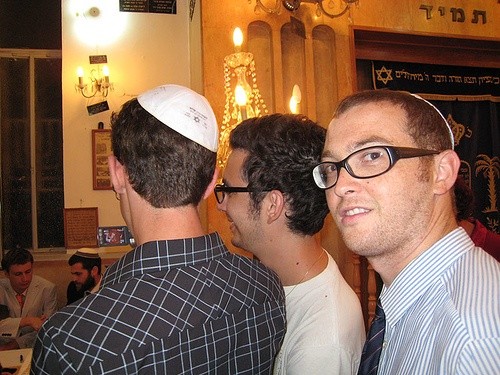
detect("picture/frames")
[99,225,128,247]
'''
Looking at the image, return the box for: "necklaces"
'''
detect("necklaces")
[285,250,324,298]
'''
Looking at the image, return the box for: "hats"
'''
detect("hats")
[75,248,100,258]
[137,84,219,153]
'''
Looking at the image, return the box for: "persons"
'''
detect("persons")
[0,249,57,340]
[29,83,286,375]
[67,248,103,304]
[311,90,500,375]
[454,177,500,262]
[106,230,115,242]
[214,113,368,375]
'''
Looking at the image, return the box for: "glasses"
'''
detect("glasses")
[312,145,441,190]
[213,183,286,205]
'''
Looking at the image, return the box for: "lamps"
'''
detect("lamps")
[75,65,111,98]
[209,23,272,169]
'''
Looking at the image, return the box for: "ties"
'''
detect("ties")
[356,298,387,375]
[16,294,23,311]
